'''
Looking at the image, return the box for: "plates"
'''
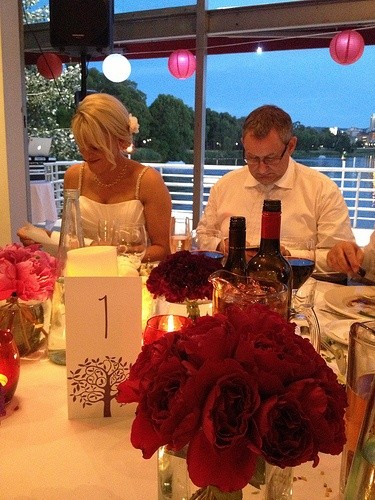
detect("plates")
[324,285,375,320]
[322,320,370,346]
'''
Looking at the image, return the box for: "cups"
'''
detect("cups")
[187,229,226,269]
[95,218,120,246]
[112,223,148,269]
[167,216,193,253]
[341,320,375,500]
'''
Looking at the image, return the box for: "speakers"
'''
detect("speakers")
[49,0,115,57]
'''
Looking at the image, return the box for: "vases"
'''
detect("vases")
[0,303,45,359]
[0,328,20,405]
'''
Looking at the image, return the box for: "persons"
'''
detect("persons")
[196,106,358,271]
[326,229,375,282]
[17,93,170,262]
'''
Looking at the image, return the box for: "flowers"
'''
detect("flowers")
[129,113,140,134]
[0,242,59,307]
[112,302,350,500]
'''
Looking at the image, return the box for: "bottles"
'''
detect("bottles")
[222,216,247,282]
[0,328,20,419]
[46,190,86,364]
[247,199,292,322]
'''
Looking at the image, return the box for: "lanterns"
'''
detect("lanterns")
[37,52,63,79]
[329,29,364,64]
[168,49,196,80]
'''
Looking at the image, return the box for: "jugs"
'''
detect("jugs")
[207,269,321,358]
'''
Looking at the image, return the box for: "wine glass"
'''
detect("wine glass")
[279,235,315,309]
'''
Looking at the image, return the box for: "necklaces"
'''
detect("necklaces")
[93,163,125,188]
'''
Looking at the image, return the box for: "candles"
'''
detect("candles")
[147,315,191,340]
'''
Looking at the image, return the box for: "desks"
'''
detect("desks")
[0,277,371,500]
[30,179,58,230]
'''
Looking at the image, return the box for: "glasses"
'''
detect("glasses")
[242,142,289,166]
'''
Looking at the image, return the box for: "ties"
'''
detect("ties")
[247,185,275,246]
